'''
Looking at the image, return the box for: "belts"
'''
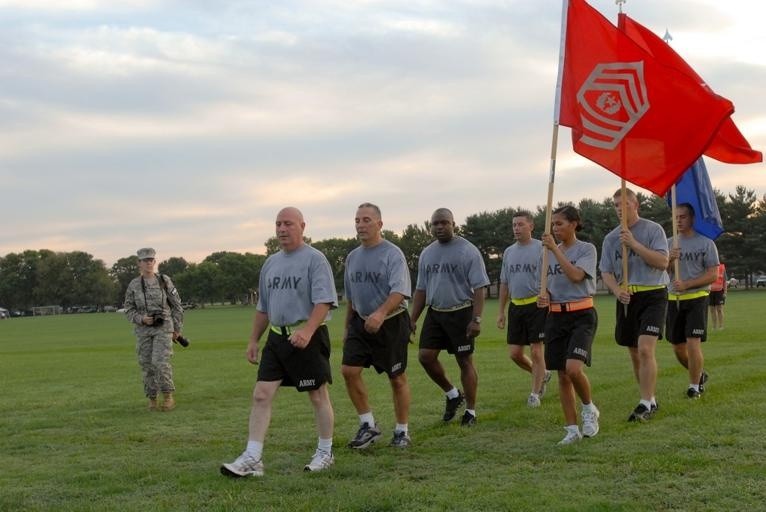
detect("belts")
[548,297,594,312]
[269,318,327,335]
[430,300,473,312]
[618,283,667,293]
[510,295,537,306]
[359,305,407,322]
[667,290,710,301]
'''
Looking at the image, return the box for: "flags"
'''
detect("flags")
[668,155,724,242]
[615,14,763,182]
[555,0,736,198]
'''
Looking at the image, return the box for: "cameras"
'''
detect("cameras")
[172,332,189,347]
[148,310,163,325]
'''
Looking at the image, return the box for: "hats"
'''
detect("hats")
[136,248,157,261]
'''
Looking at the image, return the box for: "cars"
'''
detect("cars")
[756,276,766,287]
[180,303,197,309]
[726,278,739,288]
[0,303,126,319]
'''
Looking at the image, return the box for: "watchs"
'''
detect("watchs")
[471,315,481,324]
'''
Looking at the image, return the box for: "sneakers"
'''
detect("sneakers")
[627,401,659,423]
[710,326,725,332]
[219,451,264,478]
[303,448,335,472]
[538,368,552,399]
[442,388,466,422]
[686,386,701,400]
[385,431,413,447]
[344,422,382,449]
[460,410,479,428]
[581,405,601,437]
[555,426,582,447]
[699,371,709,393]
[526,394,540,408]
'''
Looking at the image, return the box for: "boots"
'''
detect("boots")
[148,393,159,410]
[160,390,177,411]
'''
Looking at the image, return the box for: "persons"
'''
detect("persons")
[709,263,728,333]
[341,203,413,451]
[220,207,339,479]
[598,188,671,423]
[124,247,185,411]
[407,208,490,426]
[665,202,720,400]
[537,207,601,446]
[495,211,551,411]
